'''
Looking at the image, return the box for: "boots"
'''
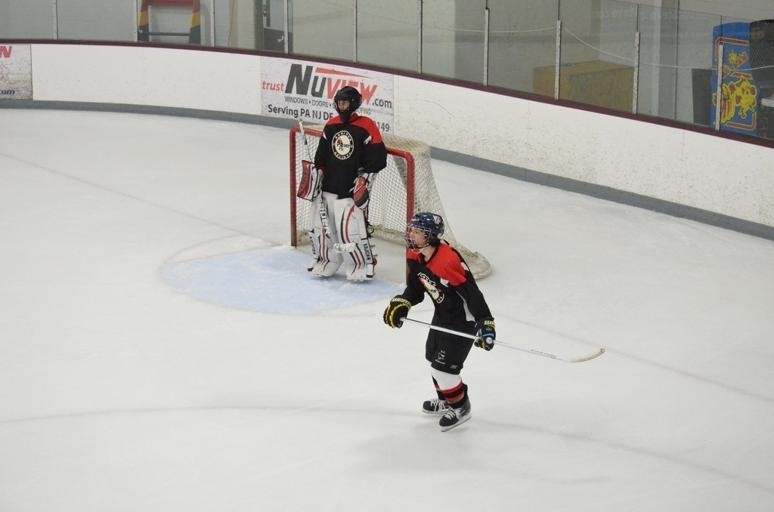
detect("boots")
[423,376,470,426]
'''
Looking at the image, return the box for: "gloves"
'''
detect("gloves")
[474,318,496,351]
[349,173,374,209]
[383,294,411,328]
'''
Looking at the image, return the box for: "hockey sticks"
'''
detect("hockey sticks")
[399,317,605,362]
[299,118,357,253]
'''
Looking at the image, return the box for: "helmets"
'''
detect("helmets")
[410,212,444,238]
[334,86,362,113]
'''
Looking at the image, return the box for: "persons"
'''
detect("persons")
[383,213,496,426]
[308,86,387,282]
